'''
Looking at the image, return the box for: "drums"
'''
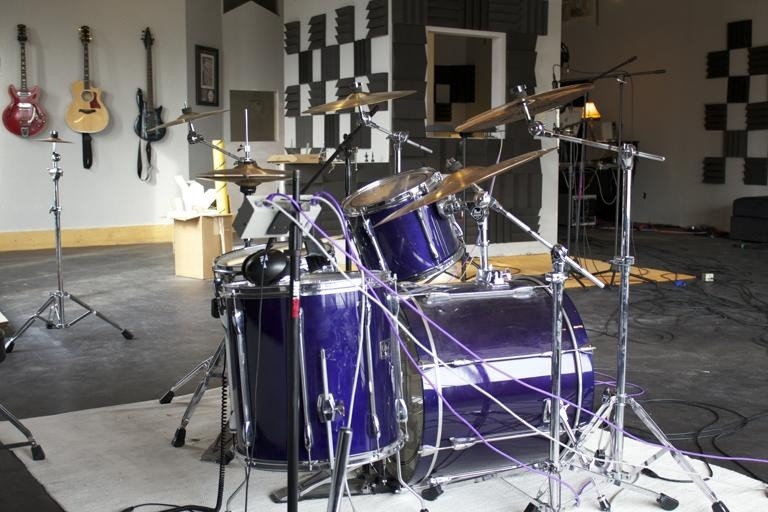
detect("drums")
[381,274,596,490]
[340,163,467,286]
[224,266,409,473]
[212,236,338,436]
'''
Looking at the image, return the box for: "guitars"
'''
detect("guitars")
[133,25,166,142]
[1,23,46,140]
[66,25,110,135]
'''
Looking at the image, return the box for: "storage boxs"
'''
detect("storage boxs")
[161,205,234,282]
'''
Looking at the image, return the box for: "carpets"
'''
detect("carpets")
[0,386,768,512]
[337,252,698,291]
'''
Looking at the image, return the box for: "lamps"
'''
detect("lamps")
[581,101,601,140]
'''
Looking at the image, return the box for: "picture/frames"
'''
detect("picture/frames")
[194,44,221,107]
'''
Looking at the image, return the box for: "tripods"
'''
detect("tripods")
[536,70,660,293]
[158,333,226,448]
[538,144,730,512]
[421,243,680,512]
[4,132,133,352]
[271,116,493,512]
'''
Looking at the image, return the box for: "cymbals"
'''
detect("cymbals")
[454,83,599,134]
[147,106,228,132]
[266,155,329,166]
[370,140,560,229]
[197,164,293,188]
[301,89,419,114]
[36,137,76,144]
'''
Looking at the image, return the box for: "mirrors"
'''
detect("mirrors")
[424,24,507,140]
